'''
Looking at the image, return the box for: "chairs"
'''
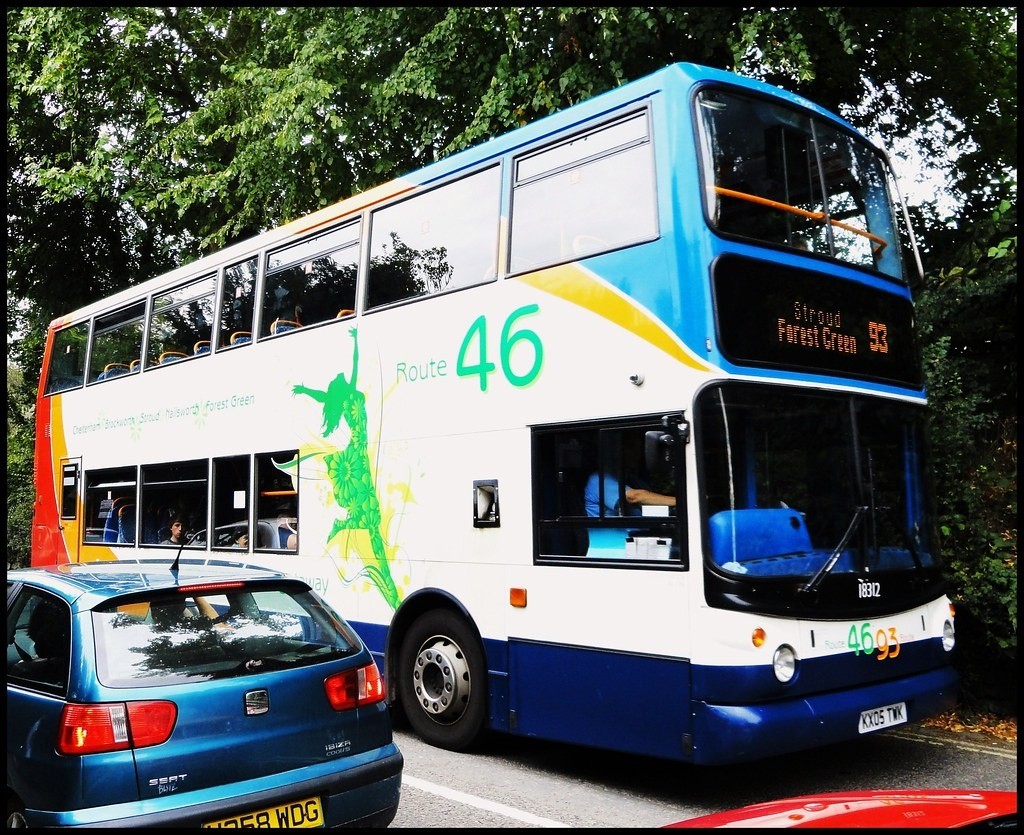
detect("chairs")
[100,495,299,552]
[97,309,361,381]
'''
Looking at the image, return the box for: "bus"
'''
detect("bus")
[28,60,963,767]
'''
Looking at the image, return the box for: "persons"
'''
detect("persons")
[581,436,676,558]
[223,529,249,548]
[19,600,64,663]
[278,514,297,548]
[161,513,191,545]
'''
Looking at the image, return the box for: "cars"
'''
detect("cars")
[655,787,1016,831]
[4,559,404,834]
